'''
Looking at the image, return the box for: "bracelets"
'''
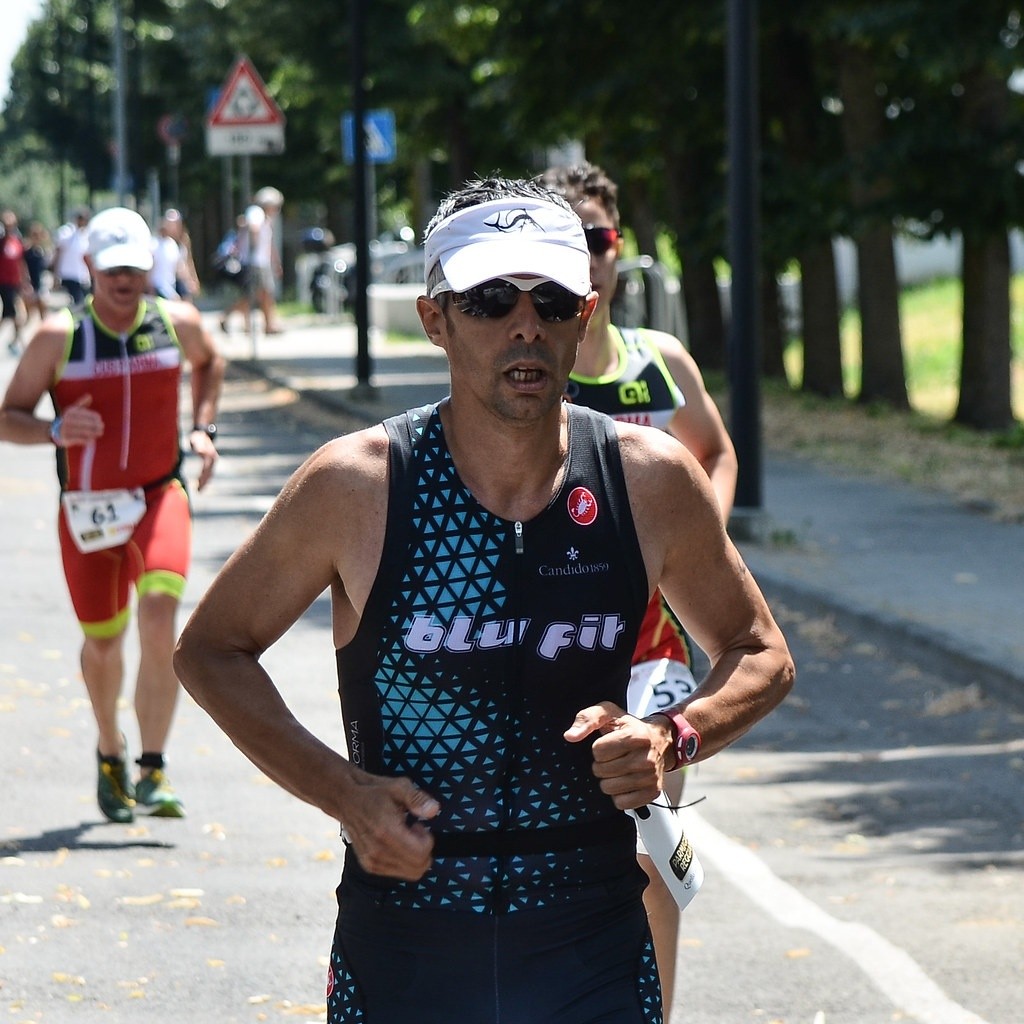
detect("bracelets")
[49,418,62,447]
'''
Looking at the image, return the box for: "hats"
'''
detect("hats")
[87,206,154,272]
[424,197,593,297]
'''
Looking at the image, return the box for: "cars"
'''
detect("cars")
[294,234,432,316]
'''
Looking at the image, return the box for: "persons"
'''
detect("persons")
[171,178,797,1024]
[0,186,289,336]
[0,205,219,821]
[535,165,735,1023]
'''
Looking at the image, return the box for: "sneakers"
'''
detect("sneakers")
[133,765,186,819]
[96,730,137,824]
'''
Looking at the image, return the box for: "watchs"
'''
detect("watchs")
[650,704,701,774]
[195,423,217,442]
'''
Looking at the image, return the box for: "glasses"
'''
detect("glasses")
[105,264,143,276]
[430,271,586,324]
[583,226,623,258]
